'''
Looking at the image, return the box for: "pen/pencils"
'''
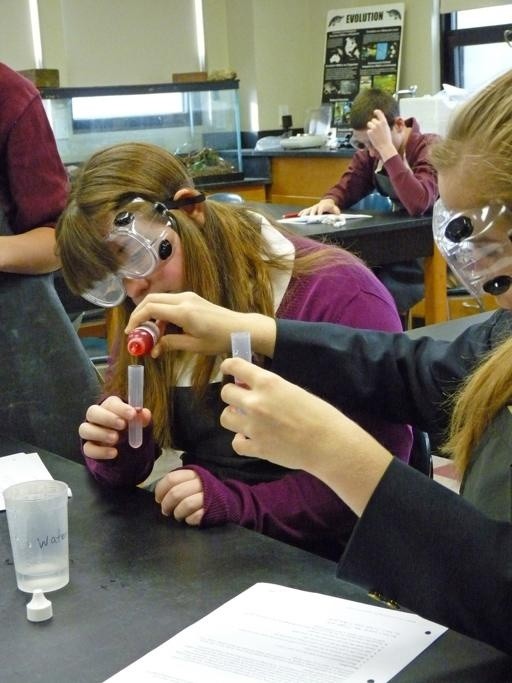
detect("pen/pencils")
[282,212,299,218]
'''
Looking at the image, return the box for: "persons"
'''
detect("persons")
[52,140,414,566]
[124,64,512,659]
[296,88,446,319]
[0,60,108,467]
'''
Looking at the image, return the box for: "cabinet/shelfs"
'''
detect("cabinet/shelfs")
[35,79,273,337]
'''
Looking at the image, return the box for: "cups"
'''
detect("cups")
[3,478,73,594]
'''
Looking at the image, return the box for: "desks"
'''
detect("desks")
[246,201,450,326]
[213,146,501,321]
[0,431,511,683]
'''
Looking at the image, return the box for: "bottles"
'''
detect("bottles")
[125,362,145,450]
[124,317,162,358]
[228,330,252,398]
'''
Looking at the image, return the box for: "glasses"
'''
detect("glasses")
[81,192,206,309]
[349,135,373,150]
[431,199,512,300]
